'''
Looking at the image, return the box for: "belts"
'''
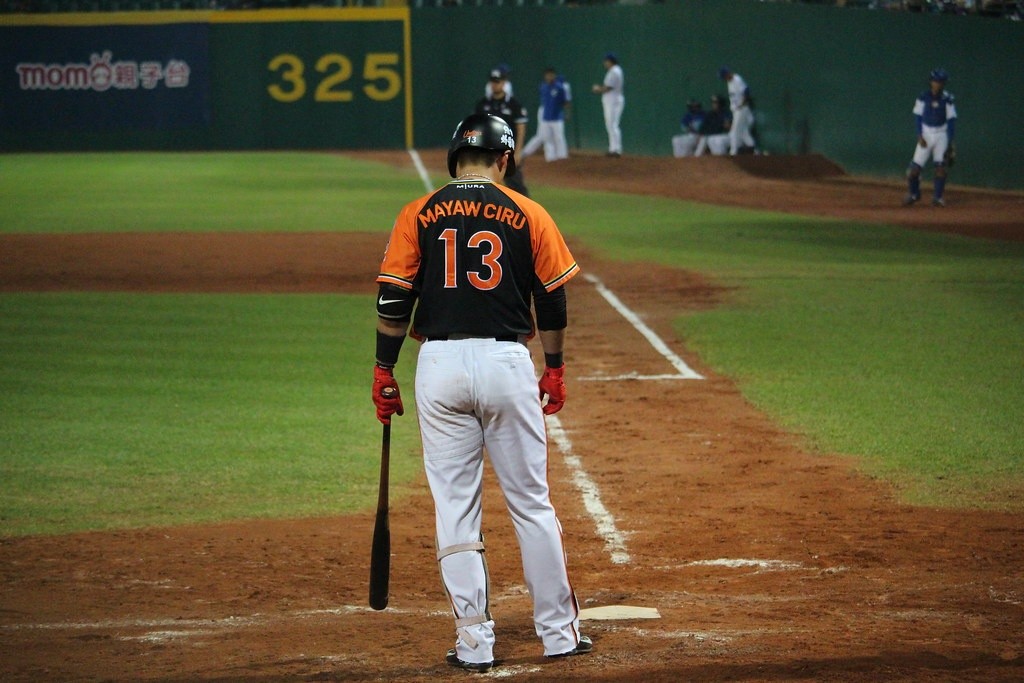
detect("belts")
[421,333,518,342]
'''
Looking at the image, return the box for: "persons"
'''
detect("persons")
[592,52,626,157]
[536,66,572,163]
[365,113,593,673]
[716,66,756,160]
[476,63,529,197]
[904,69,958,207]
[671,93,732,159]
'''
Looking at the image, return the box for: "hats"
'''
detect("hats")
[686,98,701,107]
[709,93,720,103]
[601,51,615,64]
[717,64,728,80]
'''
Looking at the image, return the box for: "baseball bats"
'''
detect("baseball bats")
[369,386,396,610]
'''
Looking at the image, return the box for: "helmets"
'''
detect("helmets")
[447,113,516,178]
[930,67,948,81]
[489,67,505,81]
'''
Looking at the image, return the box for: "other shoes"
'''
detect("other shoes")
[606,152,620,158]
[904,190,921,204]
[932,196,946,208]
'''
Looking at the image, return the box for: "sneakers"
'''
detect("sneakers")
[544,636,593,657]
[445,649,494,673]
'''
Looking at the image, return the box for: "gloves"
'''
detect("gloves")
[372,365,404,425]
[538,363,566,415]
